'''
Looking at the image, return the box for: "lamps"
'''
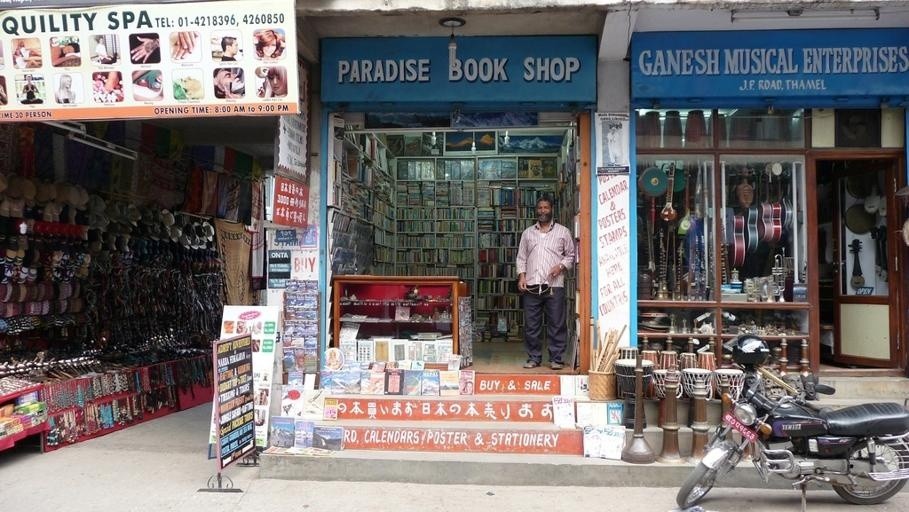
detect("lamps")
[730,7,881,23]
[439,18,469,68]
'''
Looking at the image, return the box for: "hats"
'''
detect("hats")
[1,174,216,252]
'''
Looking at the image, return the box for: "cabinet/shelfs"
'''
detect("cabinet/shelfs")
[636,300,810,379]
[326,128,579,370]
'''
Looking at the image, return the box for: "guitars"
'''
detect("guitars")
[720,163,793,267]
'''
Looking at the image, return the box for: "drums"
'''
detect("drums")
[614,346,746,428]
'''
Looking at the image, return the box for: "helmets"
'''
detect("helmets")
[732,334,769,365]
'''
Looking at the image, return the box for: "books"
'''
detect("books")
[330,122,394,276]
[563,265,580,348]
[477,218,533,278]
[478,157,558,218]
[561,138,580,243]
[397,159,475,206]
[262,279,476,456]
[396,207,474,278]
[477,280,528,343]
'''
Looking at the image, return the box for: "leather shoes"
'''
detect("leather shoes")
[550,359,563,369]
[524,358,539,368]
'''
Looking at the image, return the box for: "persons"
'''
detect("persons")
[0,29,287,103]
[606,122,623,166]
[514,196,576,369]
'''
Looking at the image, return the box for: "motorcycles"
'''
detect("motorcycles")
[677,333,909,512]
